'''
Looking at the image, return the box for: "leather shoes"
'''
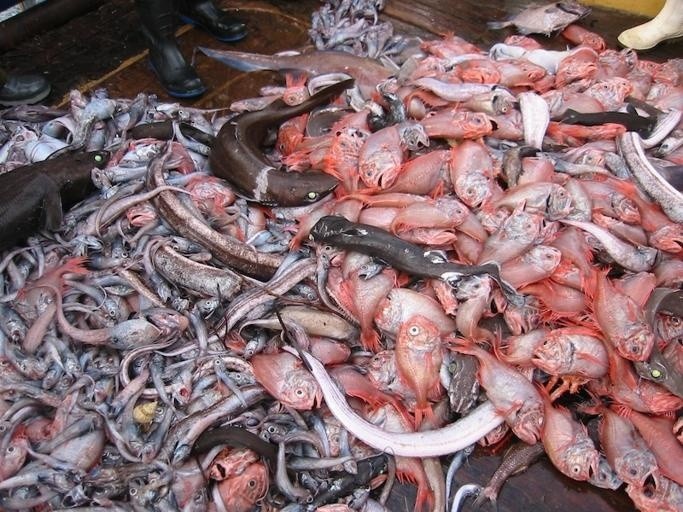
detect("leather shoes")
[0,75,51,107]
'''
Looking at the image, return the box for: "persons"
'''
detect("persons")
[0,63,53,109]
[130,0,249,101]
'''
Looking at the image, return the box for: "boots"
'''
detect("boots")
[141,0,206,98]
[179,0,248,41]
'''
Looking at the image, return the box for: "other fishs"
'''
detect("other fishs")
[0,1,683,511]
